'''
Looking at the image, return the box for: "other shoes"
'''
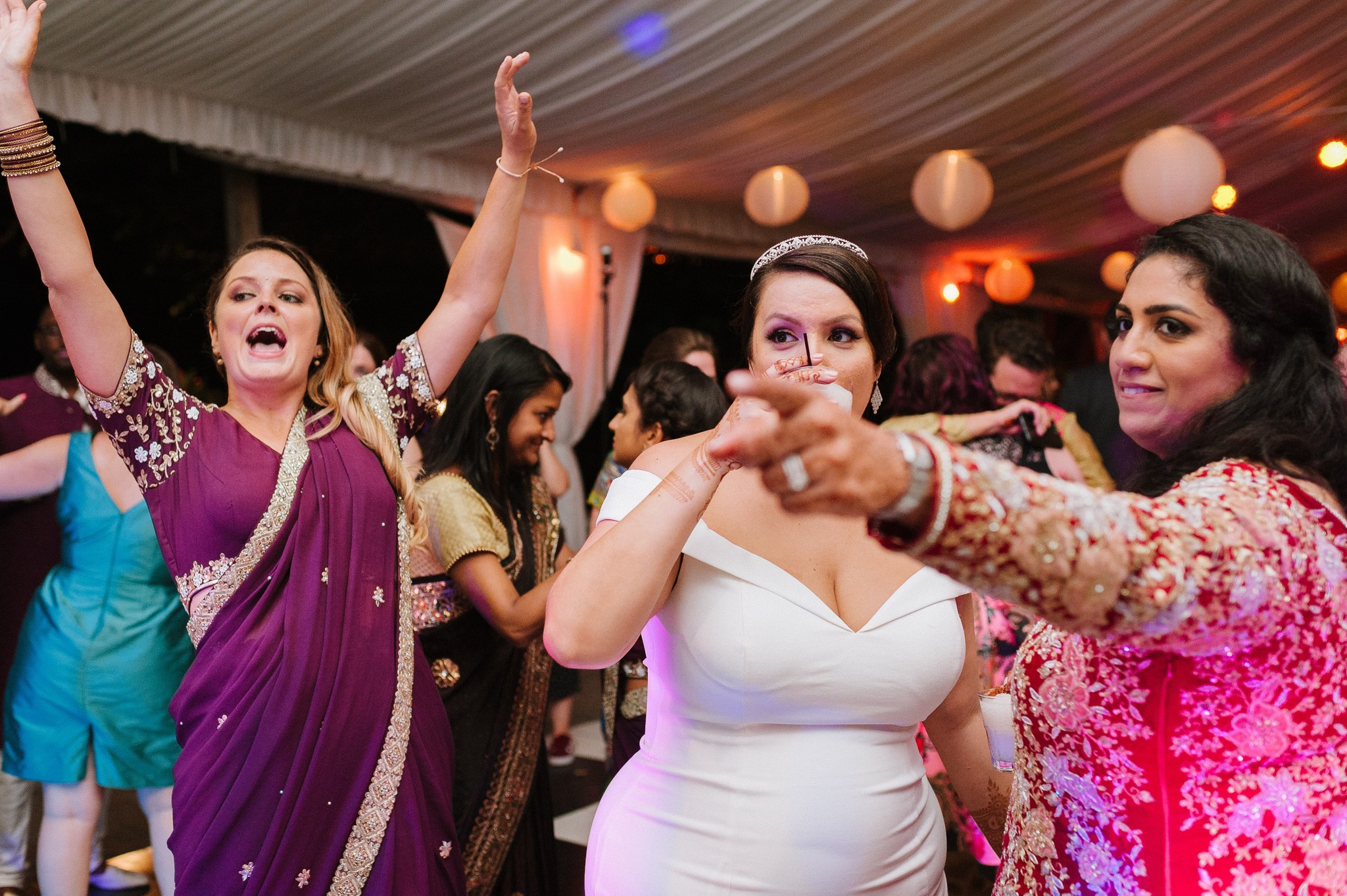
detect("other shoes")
[90,864,150,895]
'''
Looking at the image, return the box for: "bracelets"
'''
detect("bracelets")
[491,147,567,184]
[0,119,61,178]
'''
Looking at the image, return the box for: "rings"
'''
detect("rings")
[777,450,809,493]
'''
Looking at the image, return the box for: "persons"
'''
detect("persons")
[0,0,542,895]
[875,298,1118,895]
[340,329,588,895]
[0,347,206,895]
[542,238,1025,896]
[712,213,1347,896]
[2,292,158,891]
[607,358,729,784]
[863,333,1040,895]
[584,325,720,752]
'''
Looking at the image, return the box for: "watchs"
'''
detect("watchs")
[869,425,935,524]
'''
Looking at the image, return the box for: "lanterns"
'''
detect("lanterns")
[1118,121,1233,230]
[745,161,810,224]
[912,146,993,229]
[599,174,659,232]
[1100,252,1138,293]
[978,255,1037,302]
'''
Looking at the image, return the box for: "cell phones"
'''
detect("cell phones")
[1021,411,1065,448]
